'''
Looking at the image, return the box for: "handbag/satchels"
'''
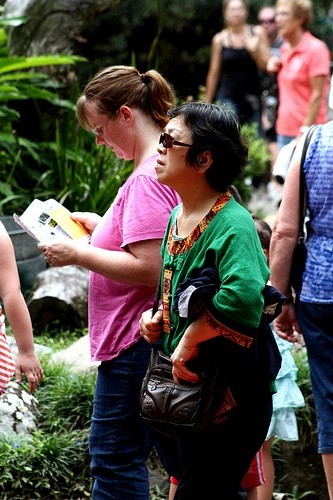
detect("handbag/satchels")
[139,348,216,427]
[288,242,307,305]
[261,85,279,141]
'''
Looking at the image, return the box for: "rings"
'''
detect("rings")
[44,256,50,263]
[140,324,145,331]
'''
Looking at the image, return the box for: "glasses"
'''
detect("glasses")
[159,133,193,148]
[91,103,125,138]
[259,19,274,23]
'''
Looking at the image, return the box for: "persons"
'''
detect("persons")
[267,73,333,500]
[0,219,44,395]
[134,100,274,500]
[36,64,183,500]
[205,0,331,154]
[167,214,298,500]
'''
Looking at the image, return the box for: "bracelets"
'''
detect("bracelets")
[282,296,294,305]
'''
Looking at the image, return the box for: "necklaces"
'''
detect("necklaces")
[228,27,234,47]
